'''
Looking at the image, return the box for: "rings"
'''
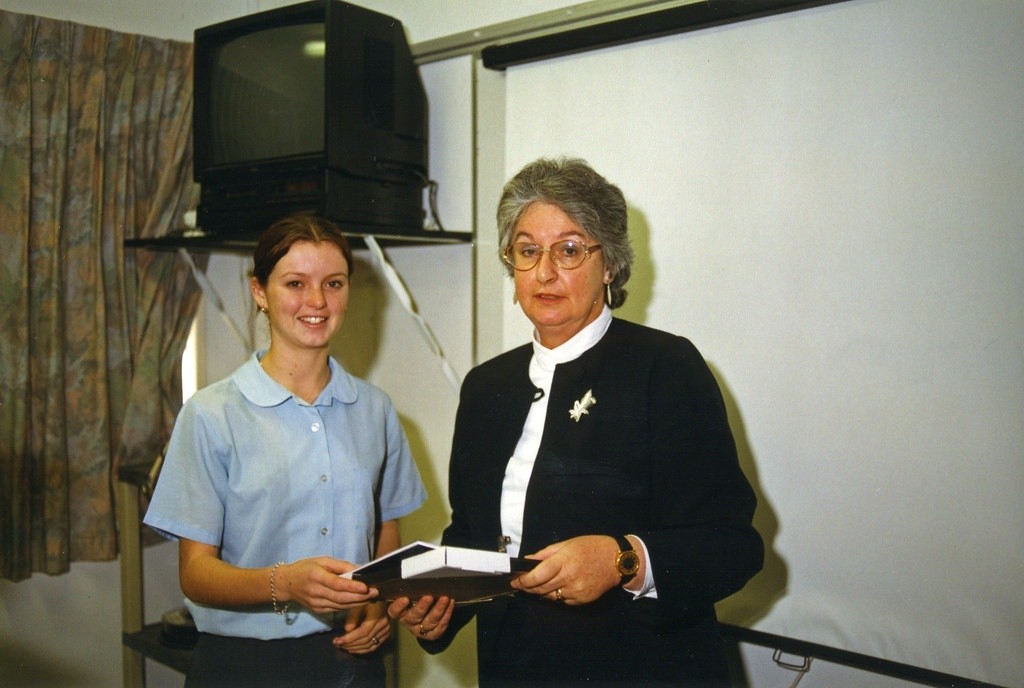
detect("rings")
[373,638,379,644]
[556,589,564,602]
[419,624,428,636]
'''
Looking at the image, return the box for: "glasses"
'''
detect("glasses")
[501,239,600,269]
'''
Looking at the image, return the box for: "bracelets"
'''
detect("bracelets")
[270,560,292,615]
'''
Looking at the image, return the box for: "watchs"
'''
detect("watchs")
[612,531,640,589]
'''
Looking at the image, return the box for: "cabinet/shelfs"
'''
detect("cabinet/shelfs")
[117,462,199,688]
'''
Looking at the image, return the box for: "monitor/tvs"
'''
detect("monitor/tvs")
[191,1,429,233]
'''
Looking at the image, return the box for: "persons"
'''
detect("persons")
[376,156,769,688]
[139,212,426,688]
